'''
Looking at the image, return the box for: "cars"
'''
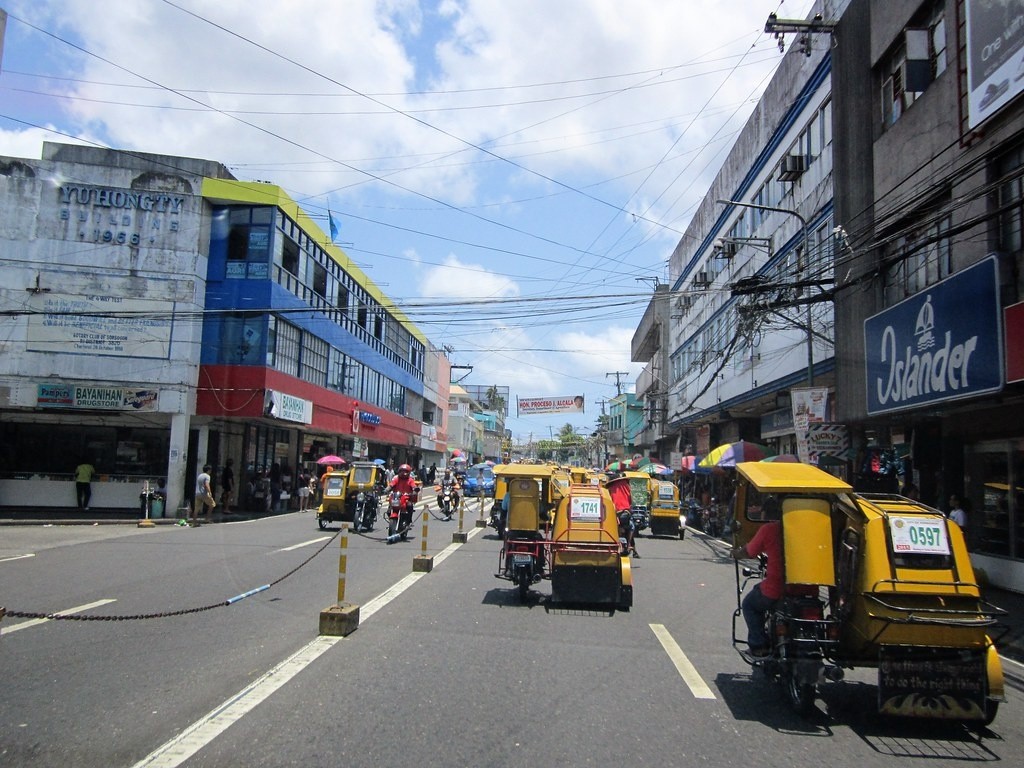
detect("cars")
[462,464,496,497]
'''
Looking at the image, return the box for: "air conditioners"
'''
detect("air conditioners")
[904,29,930,91]
[780,156,802,182]
[694,272,707,286]
[670,297,689,319]
[722,242,735,258]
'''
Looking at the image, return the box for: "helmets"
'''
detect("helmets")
[398,464,411,479]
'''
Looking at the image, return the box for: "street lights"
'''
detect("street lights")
[716,195,814,388]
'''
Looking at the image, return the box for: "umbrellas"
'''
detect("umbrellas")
[605,438,847,499]
[369,459,386,465]
[480,460,496,465]
[447,448,466,463]
[315,455,346,465]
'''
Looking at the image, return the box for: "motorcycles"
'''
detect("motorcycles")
[437,480,460,519]
[381,485,426,540]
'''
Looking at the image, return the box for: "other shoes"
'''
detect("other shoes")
[627,546,634,550]
[751,648,768,658]
[192,522,200,528]
[223,510,234,515]
[299,509,309,513]
[632,551,639,558]
[205,518,214,523]
[78,506,90,512]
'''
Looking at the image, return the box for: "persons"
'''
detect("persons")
[413,462,438,487]
[258,463,335,511]
[74,456,95,512]
[435,465,496,516]
[154,479,167,494]
[193,464,214,526]
[498,478,550,576]
[727,494,797,660]
[901,483,967,560]
[222,459,234,513]
[653,474,736,549]
[570,396,583,409]
[385,464,418,530]
[607,470,638,558]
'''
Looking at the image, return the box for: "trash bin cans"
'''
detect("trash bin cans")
[152,492,165,518]
[141,491,152,518]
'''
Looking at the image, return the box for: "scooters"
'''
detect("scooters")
[691,498,723,537]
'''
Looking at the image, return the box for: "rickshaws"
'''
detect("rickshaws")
[623,471,686,536]
[317,460,384,530]
[489,463,639,610]
[546,463,610,529]
[735,462,1008,728]
